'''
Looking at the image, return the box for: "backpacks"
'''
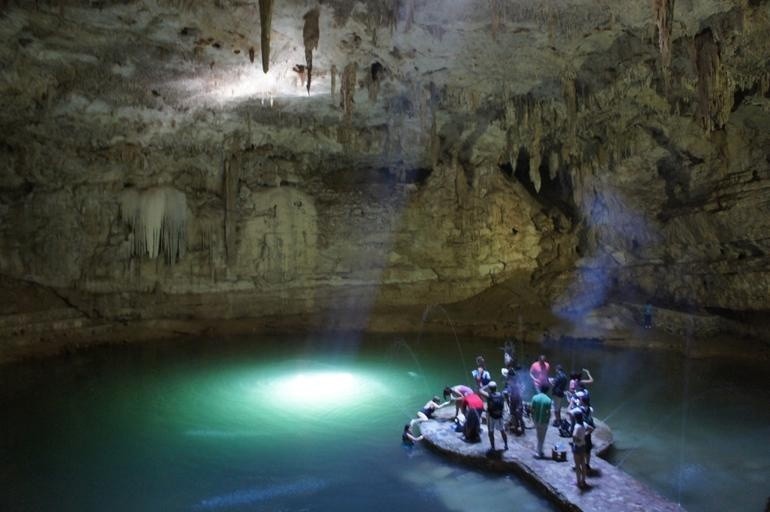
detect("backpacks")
[577,405,596,429]
[488,393,505,420]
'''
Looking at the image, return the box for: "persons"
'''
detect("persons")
[402,343,595,490]
[643,300,654,329]
